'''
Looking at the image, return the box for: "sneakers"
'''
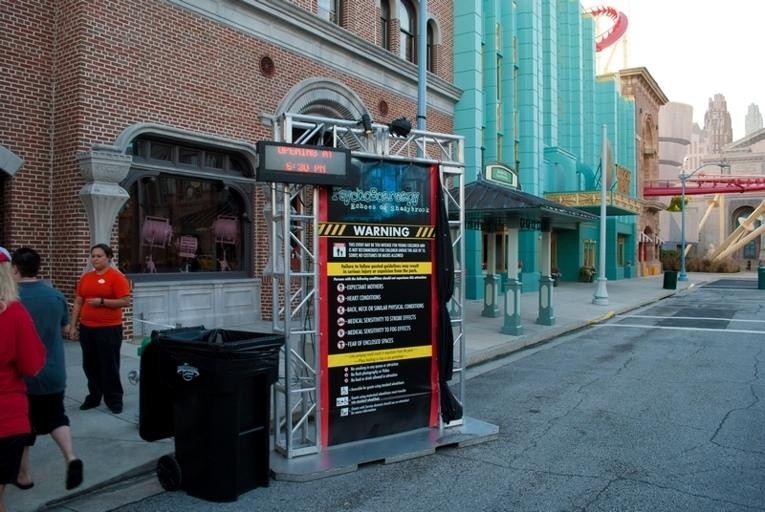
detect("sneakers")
[110,407,122,413]
[80,403,101,409]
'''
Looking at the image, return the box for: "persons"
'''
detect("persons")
[11,245,84,491]
[0,246,47,512]
[69,242,131,415]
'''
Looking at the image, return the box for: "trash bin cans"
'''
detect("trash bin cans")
[138,325,285,503]
[758,267,765,289]
[663,271,677,289]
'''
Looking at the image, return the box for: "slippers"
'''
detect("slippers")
[12,479,34,489]
[66,459,83,490]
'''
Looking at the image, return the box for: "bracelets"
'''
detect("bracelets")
[101,298,104,305]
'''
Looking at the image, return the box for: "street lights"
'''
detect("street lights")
[678,163,731,280]
